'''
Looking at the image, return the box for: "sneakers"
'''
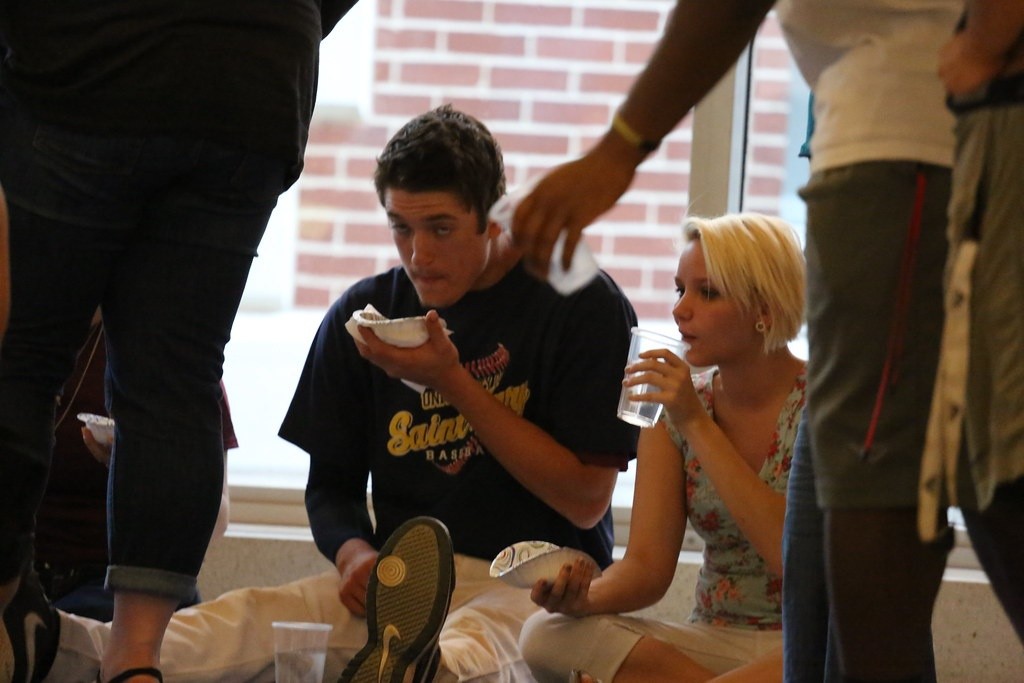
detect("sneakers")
[0,517,61,683]
[338,517,456,683]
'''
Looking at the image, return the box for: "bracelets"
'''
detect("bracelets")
[614,112,660,149]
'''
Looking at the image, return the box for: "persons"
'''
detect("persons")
[519,216,811,683]
[0,106,635,683]
[511,0,965,683]
[1,0,356,680]
[936,0,1024,646]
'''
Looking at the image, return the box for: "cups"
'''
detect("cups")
[271,622,334,683]
[617,329,692,429]
